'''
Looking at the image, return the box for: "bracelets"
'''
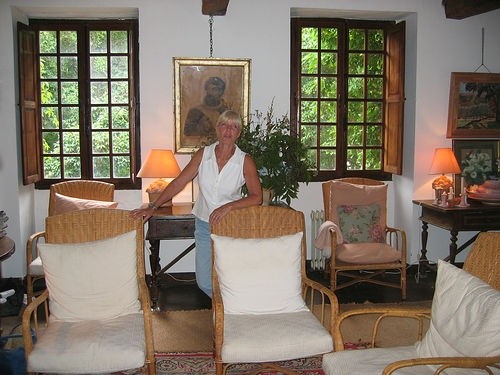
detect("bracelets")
[148,202,157,209]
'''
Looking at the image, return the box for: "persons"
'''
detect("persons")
[131,110,262,300]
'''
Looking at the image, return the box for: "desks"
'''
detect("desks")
[0,236,15,262]
[146,201,296,309]
[413,199,500,281]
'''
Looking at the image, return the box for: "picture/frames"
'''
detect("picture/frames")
[451,140,500,202]
[446,72,500,138]
[172,56,252,154]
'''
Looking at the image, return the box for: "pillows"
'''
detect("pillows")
[414,259,500,375]
[35,230,141,321]
[210,232,309,314]
[336,202,385,243]
[54,192,118,215]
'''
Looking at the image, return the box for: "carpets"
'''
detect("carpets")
[0,299,433,375]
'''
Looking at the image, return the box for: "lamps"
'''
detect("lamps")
[135,150,181,208]
[428,148,462,204]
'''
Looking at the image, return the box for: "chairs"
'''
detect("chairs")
[210,205,339,375]
[321,178,406,299]
[21,180,156,375]
[322,231,500,375]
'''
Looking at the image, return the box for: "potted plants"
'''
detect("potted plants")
[191,95,318,207]
